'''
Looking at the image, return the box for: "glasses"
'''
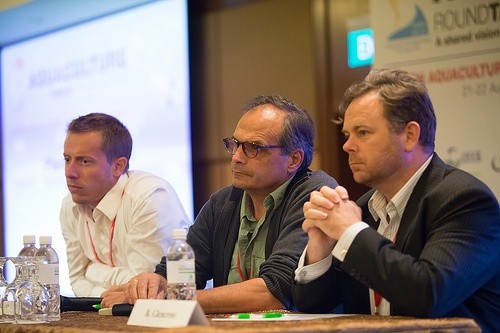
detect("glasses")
[223,137,286,159]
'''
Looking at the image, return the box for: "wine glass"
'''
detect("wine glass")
[16,256,50,324]
[2,257,22,324]
[0,256,7,324]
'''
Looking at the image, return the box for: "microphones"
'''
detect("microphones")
[60,295,103,313]
[112,304,134,316]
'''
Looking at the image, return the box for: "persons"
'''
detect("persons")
[60,113,193,297]
[100,95,340,313]
[291,69,500,333]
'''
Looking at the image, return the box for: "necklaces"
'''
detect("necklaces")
[86,172,129,267]
[237,249,252,282]
[374,229,398,315]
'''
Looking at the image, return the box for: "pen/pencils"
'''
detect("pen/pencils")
[228,312,284,319]
[92,304,101,309]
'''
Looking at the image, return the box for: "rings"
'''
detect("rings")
[128,279,138,282]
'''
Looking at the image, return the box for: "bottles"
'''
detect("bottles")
[166,229,196,301]
[34,235,60,321]
[17,235,38,322]
